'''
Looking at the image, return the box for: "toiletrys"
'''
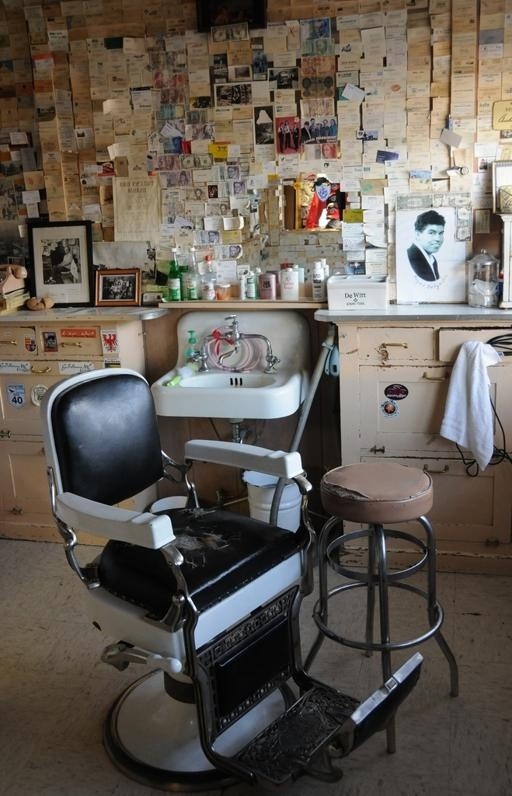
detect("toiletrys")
[184,329,198,364]
[239,261,304,300]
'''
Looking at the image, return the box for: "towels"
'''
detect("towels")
[440,339,503,473]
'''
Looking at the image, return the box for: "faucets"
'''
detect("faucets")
[225,314,241,341]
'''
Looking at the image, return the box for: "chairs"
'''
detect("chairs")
[40,366,424,793]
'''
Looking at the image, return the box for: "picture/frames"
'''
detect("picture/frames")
[27,220,143,307]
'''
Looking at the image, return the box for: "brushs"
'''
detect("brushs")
[166,366,193,387]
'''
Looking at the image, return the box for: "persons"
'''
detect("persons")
[277,118,338,153]
[407,210,445,282]
[42,241,78,284]
[103,277,135,299]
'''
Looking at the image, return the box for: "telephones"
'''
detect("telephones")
[0,264,28,294]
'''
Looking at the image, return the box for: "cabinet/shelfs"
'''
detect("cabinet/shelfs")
[0,308,247,547]
[333,321,512,576]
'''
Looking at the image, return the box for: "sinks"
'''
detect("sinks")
[152,360,310,419]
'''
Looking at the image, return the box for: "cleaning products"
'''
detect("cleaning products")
[169,246,182,301]
[187,247,202,298]
[313,257,329,301]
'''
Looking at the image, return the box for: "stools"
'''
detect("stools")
[302,462,458,699]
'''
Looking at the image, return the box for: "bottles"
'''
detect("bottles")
[167,247,231,301]
[312,261,326,304]
[280,263,299,302]
[469,249,501,308]
